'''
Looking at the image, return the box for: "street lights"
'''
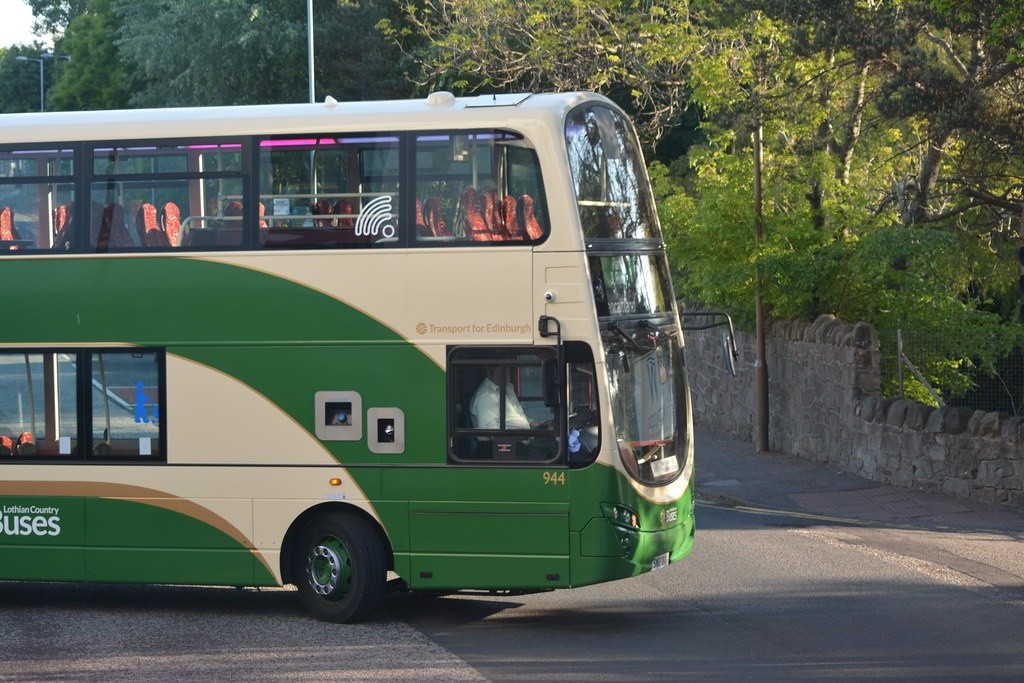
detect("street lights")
[15,56,44,113]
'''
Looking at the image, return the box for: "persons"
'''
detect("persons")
[468,355,544,459]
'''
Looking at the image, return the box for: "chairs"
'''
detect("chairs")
[0,435,14,456]
[0,206,27,250]
[416,198,432,237]
[460,188,543,242]
[160,201,185,247]
[311,200,335,228]
[423,197,453,237]
[54,205,67,239]
[15,431,41,456]
[258,201,268,229]
[223,200,243,228]
[135,203,167,247]
[91,200,115,247]
[460,355,487,459]
[99,202,135,247]
[333,200,355,227]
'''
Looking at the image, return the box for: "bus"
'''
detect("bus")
[0,90,741,626]
